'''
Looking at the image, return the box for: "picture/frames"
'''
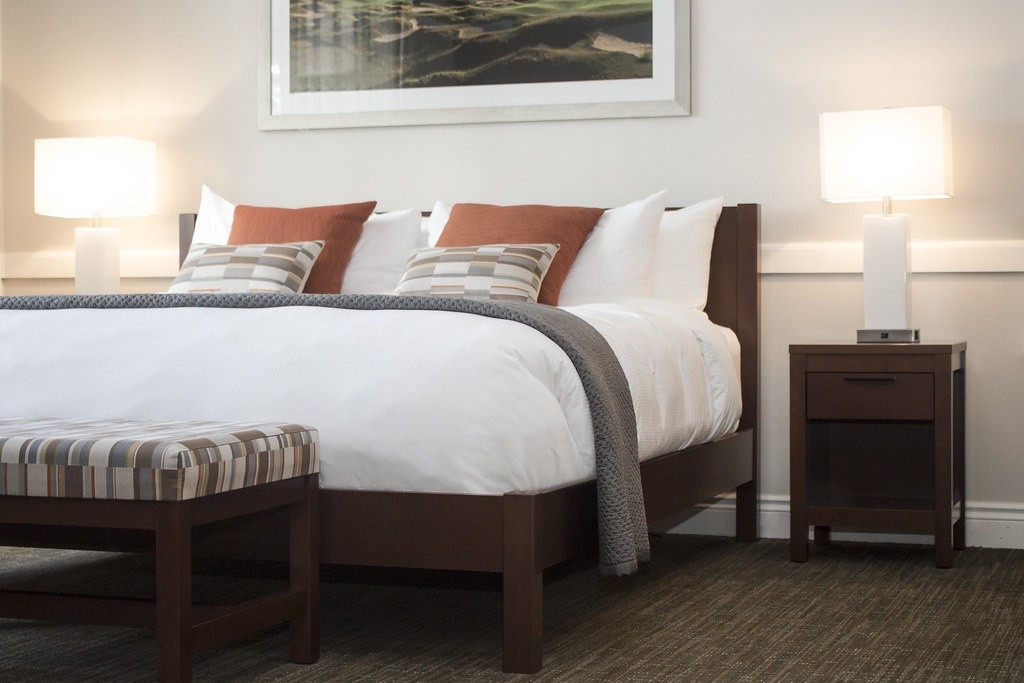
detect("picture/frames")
[259,0,693,132]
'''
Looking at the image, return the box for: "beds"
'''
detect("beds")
[0,202,761,674]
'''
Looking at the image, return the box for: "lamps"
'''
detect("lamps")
[33,135,159,295]
[819,104,954,343]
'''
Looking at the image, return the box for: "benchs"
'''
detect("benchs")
[0,419,322,683]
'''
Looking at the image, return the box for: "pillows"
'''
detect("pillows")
[226,200,376,293]
[436,203,607,305]
[394,243,561,302]
[427,187,670,303]
[168,240,326,294]
[191,184,422,297]
[646,194,726,311]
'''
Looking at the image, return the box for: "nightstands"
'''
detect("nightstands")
[787,342,970,571]
